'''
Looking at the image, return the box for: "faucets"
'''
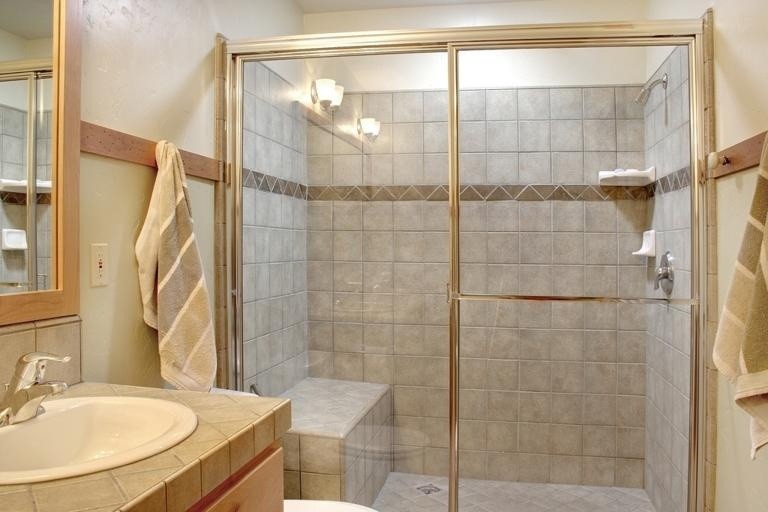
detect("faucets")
[4,351,73,422]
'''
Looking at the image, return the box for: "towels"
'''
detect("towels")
[713,138,768,460]
[135,141,218,391]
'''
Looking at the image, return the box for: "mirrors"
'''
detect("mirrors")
[0,0,82,333]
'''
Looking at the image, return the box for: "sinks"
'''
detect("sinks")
[1,394,200,485]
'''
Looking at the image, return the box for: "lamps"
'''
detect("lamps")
[358,116,380,142]
[311,78,344,110]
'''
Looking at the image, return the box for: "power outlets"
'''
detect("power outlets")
[89,243,108,288]
